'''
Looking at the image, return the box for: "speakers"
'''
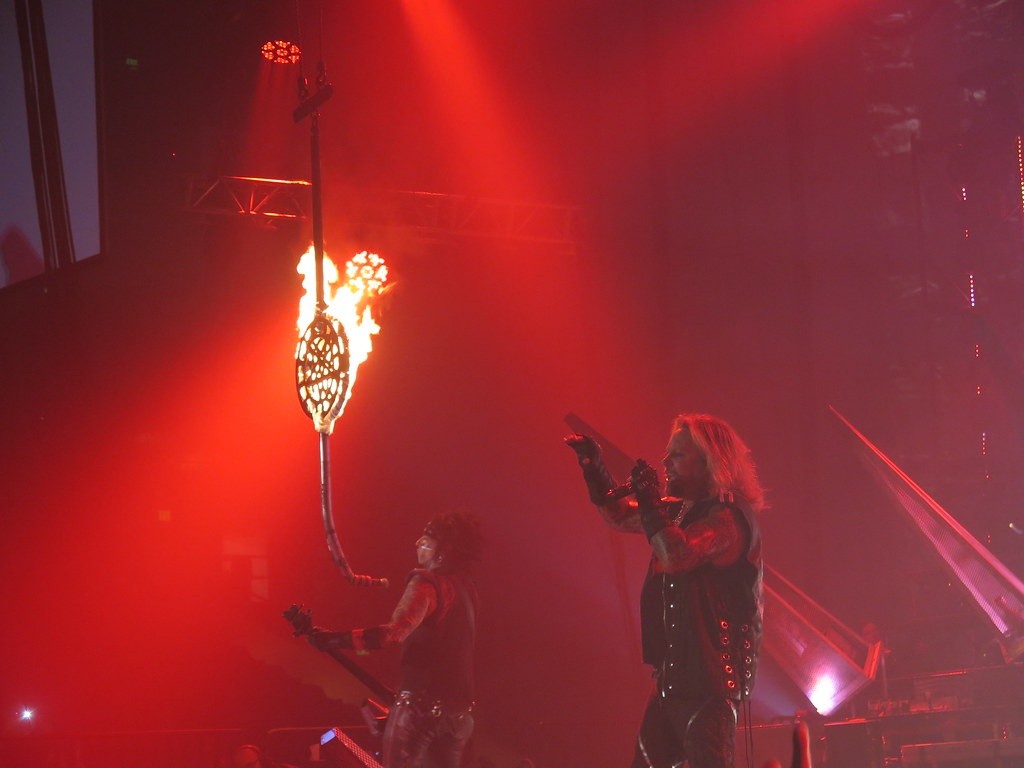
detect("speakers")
[319,727,383,768]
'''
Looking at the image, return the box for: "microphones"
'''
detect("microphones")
[606,481,635,501]
[351,576,390,589]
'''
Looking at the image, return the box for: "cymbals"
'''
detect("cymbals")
[823,717,873,728]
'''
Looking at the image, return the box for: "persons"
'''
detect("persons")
[563,414,765,768]
[307,508,486,768]
[851,623,890,713]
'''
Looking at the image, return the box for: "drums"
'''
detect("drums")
[971,592,1024,665]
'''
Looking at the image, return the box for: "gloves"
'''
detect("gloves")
[563,433,618,506]
[309,626,352,650]
[632,458,674,543]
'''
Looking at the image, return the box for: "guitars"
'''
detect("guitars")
[281,602,398,724]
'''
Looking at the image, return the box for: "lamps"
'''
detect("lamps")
[257,1,302,65]
[793,711,825,768]
